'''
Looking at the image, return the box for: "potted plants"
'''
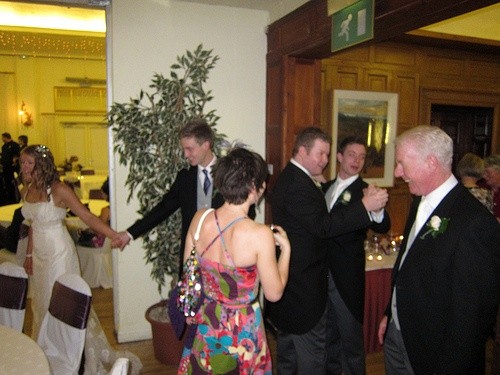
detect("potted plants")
[105,45,228,365]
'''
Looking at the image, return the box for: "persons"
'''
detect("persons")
[0,120,392,375]
[378,123,500,375]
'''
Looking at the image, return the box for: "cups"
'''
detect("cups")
[366,235,405,262]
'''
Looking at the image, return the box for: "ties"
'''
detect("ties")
[414,200,427,236]
[202,169,211,196]
[330,182,344,210]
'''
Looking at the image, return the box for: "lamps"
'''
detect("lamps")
[19,101,33,126]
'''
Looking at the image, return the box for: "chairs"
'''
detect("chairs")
[37,273,92,375]
[0,262,28,332]
[89,189,108,200]
[76,236,114,289]
[0,219,30,266]
[82,170,96,176]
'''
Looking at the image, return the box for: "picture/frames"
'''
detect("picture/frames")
[330,89,398,187]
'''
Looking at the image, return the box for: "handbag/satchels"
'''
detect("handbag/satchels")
[177,208,214,318]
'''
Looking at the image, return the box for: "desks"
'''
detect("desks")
[0,199,113,289]
[363,250,399,356]
[0,325,53,375]
[60,174,107,199]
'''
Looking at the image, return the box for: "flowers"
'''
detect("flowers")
[420,215,451,240]
[341,190,351,205]
